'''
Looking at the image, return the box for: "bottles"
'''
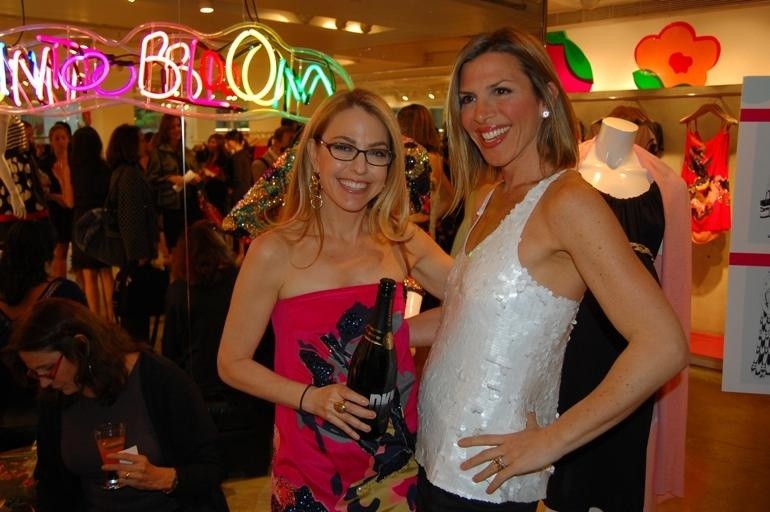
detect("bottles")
[345,277,397,442]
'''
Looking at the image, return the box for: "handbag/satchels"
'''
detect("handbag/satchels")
[88,168,125,266]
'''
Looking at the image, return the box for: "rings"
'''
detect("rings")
[491,456,506,471]
[334,399,348,414]
[126,471,130,479]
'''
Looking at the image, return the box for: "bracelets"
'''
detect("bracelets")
[300,384,313,411]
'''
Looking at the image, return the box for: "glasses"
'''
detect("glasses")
[27,354,64,379]
[316,136,394,167]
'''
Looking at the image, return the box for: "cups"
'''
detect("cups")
[97,423,127,489]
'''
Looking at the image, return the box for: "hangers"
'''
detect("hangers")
[679,92,739,127]
[590,93,665,137]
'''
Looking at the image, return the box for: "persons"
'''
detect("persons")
[405,22,688,512]
[1,99,36,218]
[224,129,251,195]
[106,123,159,344]
[398,103,455,223]
[0,216,91,450]
[280,112,298,141]
[217,89,456,511]
[40,124,86,294]
[145,114,202,251]
[198,134,238,215]
[44,126,118,325]
[17,297,229,512]
[21,118,35,163]
[267,127,290,156]
